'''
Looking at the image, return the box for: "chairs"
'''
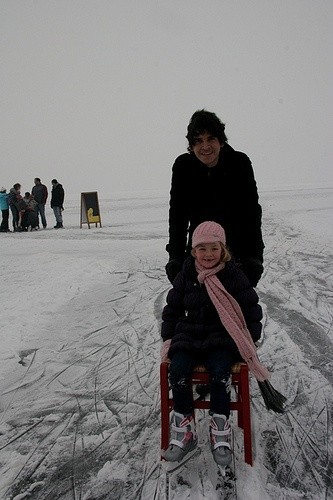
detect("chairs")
[160,358,253,467]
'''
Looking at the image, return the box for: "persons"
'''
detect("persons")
[161,221,287,476]
[165,110,265,397]
[0,178,64,233]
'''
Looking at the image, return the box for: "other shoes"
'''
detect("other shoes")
[15,228,23,231]
[54,222,62,228]
[28,225,31,232]
[2,228,10,232]
[36,226,40,231]
[43,224,46,228]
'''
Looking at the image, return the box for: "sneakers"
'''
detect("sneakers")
[167,413,199,472]
[211,415,232,476]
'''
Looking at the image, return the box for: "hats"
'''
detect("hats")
[53,180,57,183]
[1,187,6,191]
[192,221,226,248]
